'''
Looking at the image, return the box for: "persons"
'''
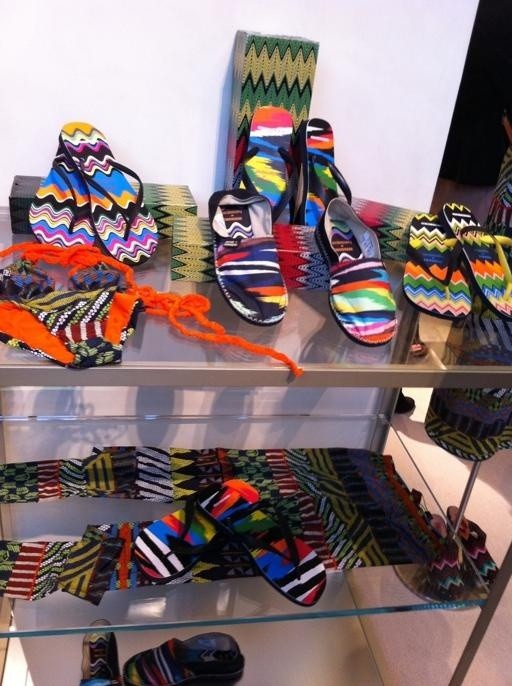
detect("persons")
[393,388,416,414]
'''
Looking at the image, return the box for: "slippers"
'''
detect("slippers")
[131,479,328,607]
[403,202,512,321]
[233,105,352,225]
[28,122,159,267]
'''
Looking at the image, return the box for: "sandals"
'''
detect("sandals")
[72,618,243,685]
[428,505,498,601]
[316,197,400,348]
[206,186,291,325]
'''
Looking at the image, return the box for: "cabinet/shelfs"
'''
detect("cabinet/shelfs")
[0,232,512,685]
